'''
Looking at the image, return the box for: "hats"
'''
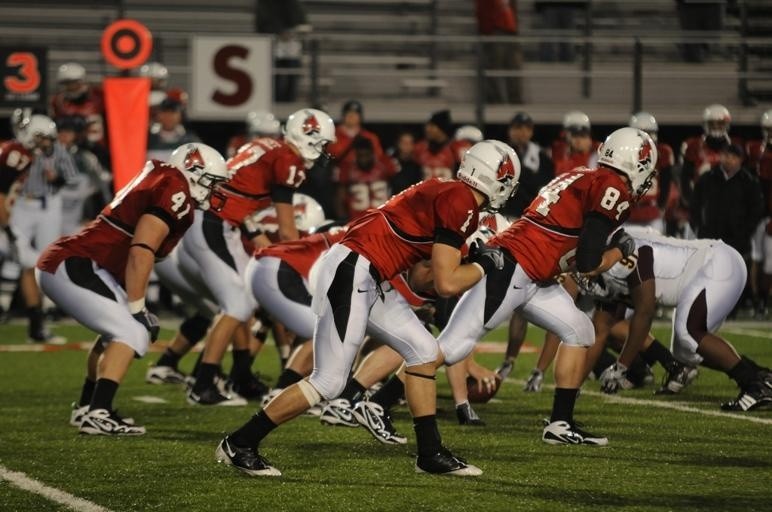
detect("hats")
[428,109,453,135]
[159,97,180,111]
[343,99,362,113]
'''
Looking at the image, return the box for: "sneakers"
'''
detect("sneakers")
[541,418,608,446]
[652,361,700,396]
[415,446,483,476]
[186,375,249,407]
[144,361,186,385]
[259,389,323,416]
[229,373,270,399]
[69,401,134,426]
[525,367,545,392]
[351,398,409,446]
[494,359,516,379]
[319,399,361,428]
[720,368,772,411]
[214,432,282,477]
[78,408,146,437]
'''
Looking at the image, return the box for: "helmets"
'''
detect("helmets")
[56,62,88,99]
[456,139,522,214]
[291,191,326,236]
[140,61,169,89]
[760,109,772,145]
[455,125,483,142]
[561,111,590,133]
[629,111,658,137]
[702,103,731,139]
[16,114,58,156]
[283,107,339,168]
[597,126,659,203]
[167,85,191,106]
[168,142,233,212]
[240,111,281,143]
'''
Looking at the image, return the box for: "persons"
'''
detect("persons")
[345,126,658,449]
[748,218,772,313]
[628,111,674,183]
[33,142,227,435]
[745,110,772,187]
[143,193,326,385]
[139,62,188,123]
[414,109,471,182]
[325,100,395,218]
[492,276,698,396]
[213,139,522,476]
[388,130,423,194]
[689,145,763,320]
[568,225,772,413]
[1,123,32,319]
[678,103,747,208]
[183,107,339,407]
[550,110,601,177]
[146,97,201,165]
[502,113,552,214]
[52,62,104,141]
[243,209,511,426]
[12,114,80,342]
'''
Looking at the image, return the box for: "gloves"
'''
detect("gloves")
[128,297,161,345]
[464,241,479,263]
[598,361,629,394]
[472,237,505,276]
[610,226,635,258]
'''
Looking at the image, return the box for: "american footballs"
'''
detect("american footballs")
[463,374,502,406]
